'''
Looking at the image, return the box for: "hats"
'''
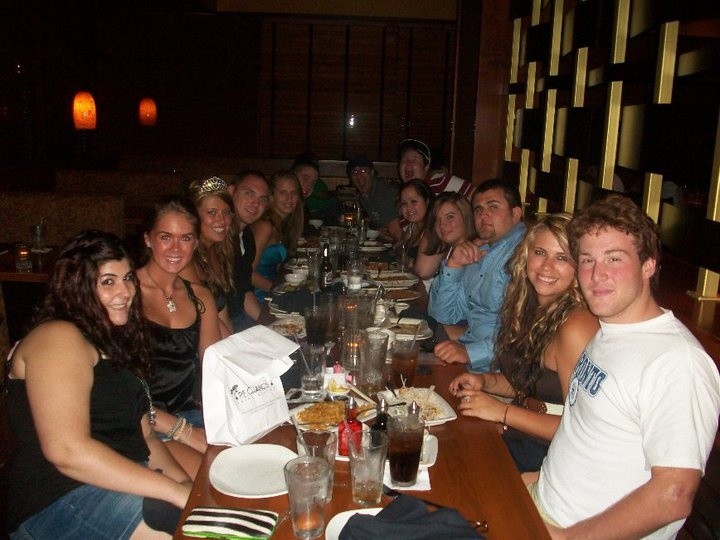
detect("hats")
[396,139,430,163]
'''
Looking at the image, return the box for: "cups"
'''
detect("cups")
[30,224,48,248]
[282,250,455,539]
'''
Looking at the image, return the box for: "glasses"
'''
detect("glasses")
[351,168,372,174]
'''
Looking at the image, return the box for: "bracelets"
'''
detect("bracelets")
[175,419,193,442]
[166,417,183,438]
[503,403,512,425]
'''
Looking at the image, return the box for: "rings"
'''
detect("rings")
[465,396,470,402]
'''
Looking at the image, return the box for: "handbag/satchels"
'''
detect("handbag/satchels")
[201,325,300,448]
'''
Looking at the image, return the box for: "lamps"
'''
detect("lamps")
[71,90,97,130]
[138,97,158,127]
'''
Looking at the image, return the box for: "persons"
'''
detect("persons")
[134,140,600,485]
[0,229,195,540]
[521,194,719,540]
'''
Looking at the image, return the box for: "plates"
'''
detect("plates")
[377,317,428,337]
[284,258,309,268]
[384,289,422,301]
[209,444,298,499]
[298,432,439,466]
[297,247,322,253]
[359,246,386,252]
[395,316,433,340]
[268,318,307,339]
[288,397,377,432]
[268,301,300,314]
[377,386,460,427]
[267,310,301,319]
[367,272,419,286]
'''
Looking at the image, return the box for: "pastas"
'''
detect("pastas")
[297,400,376,432]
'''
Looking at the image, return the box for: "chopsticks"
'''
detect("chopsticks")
[347,381,379,406]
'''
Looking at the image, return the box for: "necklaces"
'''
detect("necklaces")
[145,266,176,312]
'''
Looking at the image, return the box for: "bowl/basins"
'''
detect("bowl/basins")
[285,274,307,286]
[395,303,410,313]
[292,266,309,275]
[367,230,380,240]
[326,385,351,394]
[309,219,322,228]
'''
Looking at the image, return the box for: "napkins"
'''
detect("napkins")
[382,460,432,492]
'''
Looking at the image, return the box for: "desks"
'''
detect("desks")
[0,241,64,393]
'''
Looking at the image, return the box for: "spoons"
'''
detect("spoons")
[388,310,407,329]
[286,390,302,401]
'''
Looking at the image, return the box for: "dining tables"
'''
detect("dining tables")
[172,226,553,540]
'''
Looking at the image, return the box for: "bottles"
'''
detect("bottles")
[352,199,366,243]
[337,250,350,294]
[318,244,333,292]
